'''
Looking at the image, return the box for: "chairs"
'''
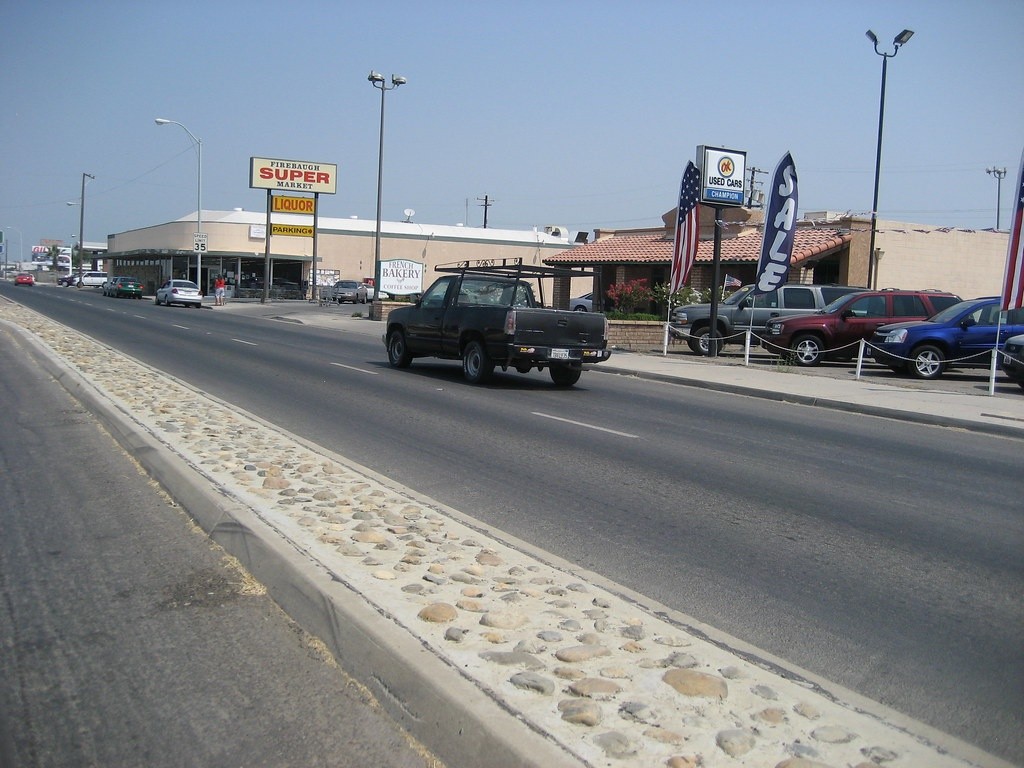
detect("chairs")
[992,310,1003,324]
[500,288,518,304]
[458,292,470,302]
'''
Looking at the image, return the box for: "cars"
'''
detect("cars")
[155,280,202,307]
[872,294,1024,378]
[15,273,35,285]
[102,277,144,299]
[329,279,368,303]
[57,274,80,287]
[569,291,592,312]
[362,282,389,301]
[72,270,108,288]
[1001,332,1023,392]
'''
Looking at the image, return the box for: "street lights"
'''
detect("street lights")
[8,226,21,273]
[368,71,407,299]
[985,166,1008,230]
[155,119,202,292]
[865,28,915,284]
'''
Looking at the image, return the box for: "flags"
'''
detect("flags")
[724,274,742,286]
[671,160,701,296]
[998,147,1024,313]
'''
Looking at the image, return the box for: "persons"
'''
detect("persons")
[214,274,225,306]
[30,273,35,284]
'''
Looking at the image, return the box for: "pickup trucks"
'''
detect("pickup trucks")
[383,275,615,390]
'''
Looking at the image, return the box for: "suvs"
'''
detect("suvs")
[670,284,879,355]
[761,290,968,364]
